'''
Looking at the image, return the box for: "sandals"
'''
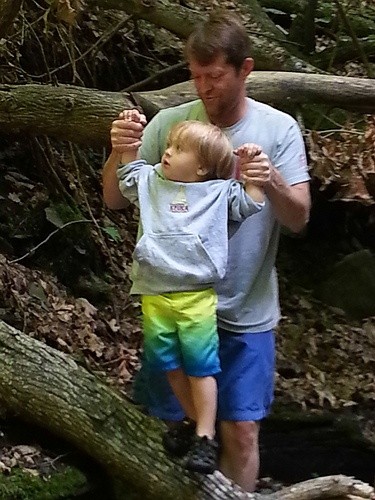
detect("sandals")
[163,417,198,457]
[185,436,220,474]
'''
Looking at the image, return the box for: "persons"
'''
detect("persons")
[111,109,270,474]
[101,0,316,493]
